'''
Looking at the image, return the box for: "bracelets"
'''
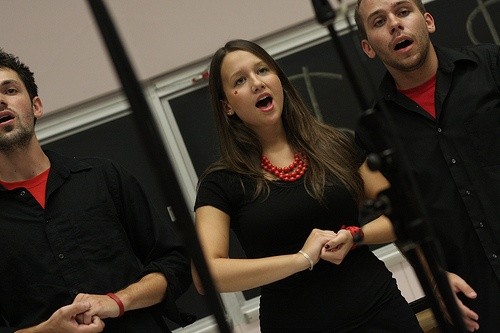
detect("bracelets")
[106,292,125,318]
[299,250,313,271]
[340,225,364,249]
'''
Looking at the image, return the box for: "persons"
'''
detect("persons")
[192,39,424,333]
[345,0,500,333]
[0,51,194,333]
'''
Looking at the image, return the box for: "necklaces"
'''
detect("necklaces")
[260,151,309,182]
